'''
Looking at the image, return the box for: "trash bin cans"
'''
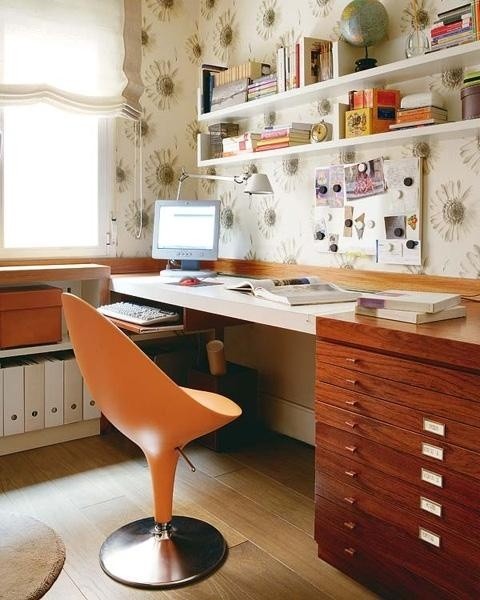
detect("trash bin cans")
[187,357,257,453]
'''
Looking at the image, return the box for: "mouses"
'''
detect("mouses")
[180,277,201,286]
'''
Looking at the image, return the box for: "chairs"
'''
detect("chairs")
[60,291,245,592]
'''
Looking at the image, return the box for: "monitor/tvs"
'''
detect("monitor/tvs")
[152,199,221,277]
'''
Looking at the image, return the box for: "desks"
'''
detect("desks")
[101,271,480,600]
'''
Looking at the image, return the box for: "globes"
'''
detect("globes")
[339,0,388,72]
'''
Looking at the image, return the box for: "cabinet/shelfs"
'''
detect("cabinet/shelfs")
[194,39,480,165]
[1,264,111,457]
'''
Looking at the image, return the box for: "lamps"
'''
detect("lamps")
[158,163,275,279]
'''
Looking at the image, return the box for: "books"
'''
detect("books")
[356,287,468,325]
[226,275,361,306]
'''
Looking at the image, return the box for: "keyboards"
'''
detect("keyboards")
[96,301,180,326]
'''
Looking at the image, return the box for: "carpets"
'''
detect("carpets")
[1,510,66,600]
